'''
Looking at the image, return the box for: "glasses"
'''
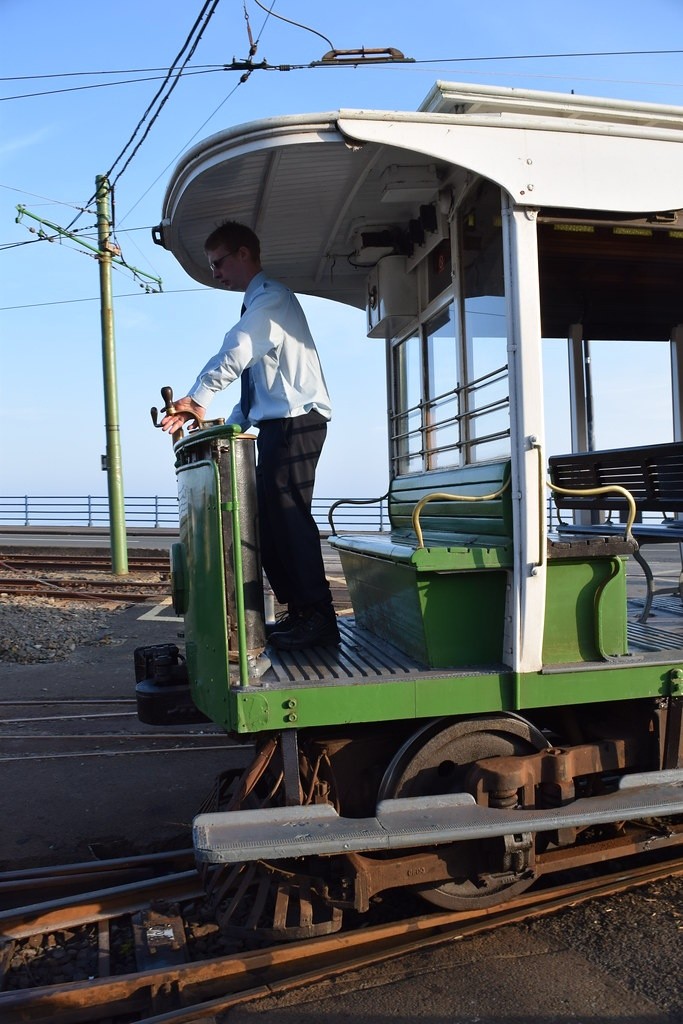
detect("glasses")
[208,252,233,272]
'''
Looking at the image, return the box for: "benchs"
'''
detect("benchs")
[329,465,643,662]
[547,441,683,634]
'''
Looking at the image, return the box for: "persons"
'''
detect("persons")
[161,218,341,653]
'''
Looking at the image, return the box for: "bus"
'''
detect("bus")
[121,72,682,929]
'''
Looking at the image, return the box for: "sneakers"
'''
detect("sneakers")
[265,604,343,650]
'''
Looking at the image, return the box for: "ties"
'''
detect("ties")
[240,302,251,420]
